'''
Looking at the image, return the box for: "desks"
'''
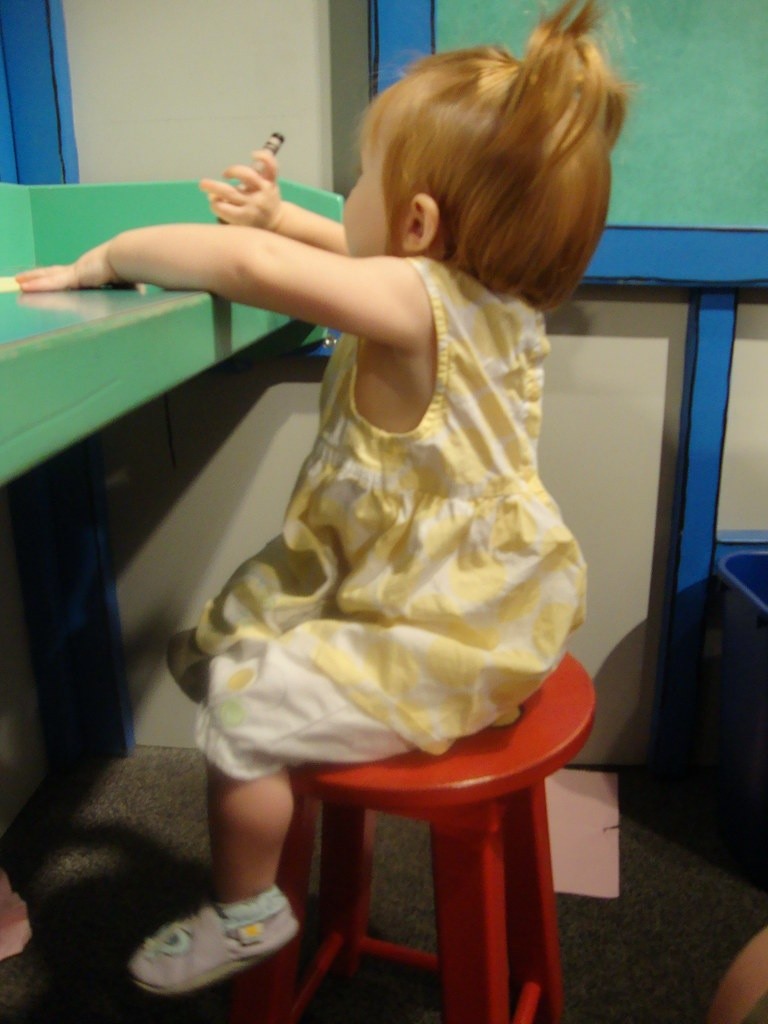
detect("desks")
[0,179,347,766]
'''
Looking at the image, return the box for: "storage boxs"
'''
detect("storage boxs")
[714,550,768,890]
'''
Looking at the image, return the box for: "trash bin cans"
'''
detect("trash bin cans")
[711,549,768,892]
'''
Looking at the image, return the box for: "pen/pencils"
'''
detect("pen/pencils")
[216,132,285,226]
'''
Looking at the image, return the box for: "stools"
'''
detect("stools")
[231,650,595,1024]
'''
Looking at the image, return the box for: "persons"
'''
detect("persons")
[11,1,629,1000]
